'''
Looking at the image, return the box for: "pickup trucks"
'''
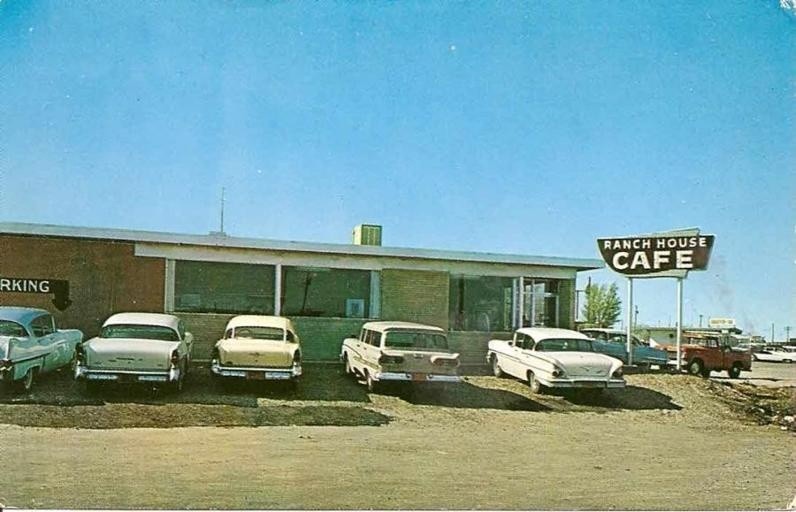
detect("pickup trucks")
[651,328,753,378]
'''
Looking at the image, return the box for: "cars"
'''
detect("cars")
[0,305,85,396]
[209,314,305,392]
[338,321,464,393]
[730,334,796,364]
[486,327,627,396]
[69,312,195,394]
[580,327,668,374]
